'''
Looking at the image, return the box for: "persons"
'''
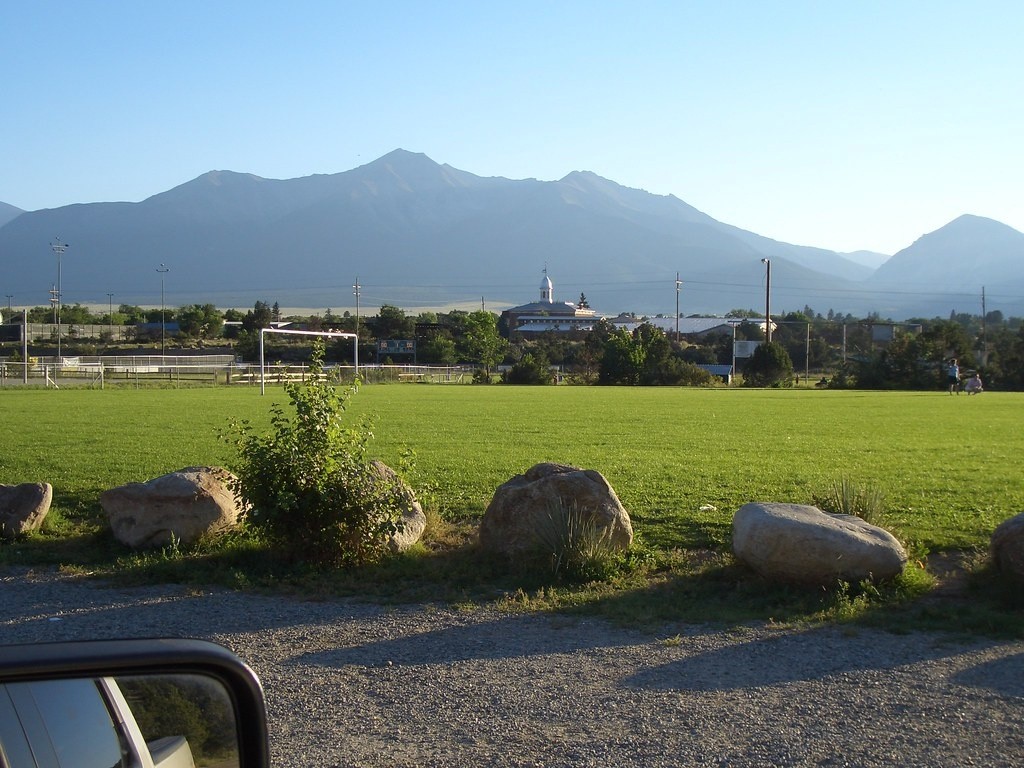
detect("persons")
[965,374,983,396]
[820,377,827,385]
[795,375,799,384]
[947,359,960,396]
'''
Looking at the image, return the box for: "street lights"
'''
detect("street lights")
[762,258,771,343]
[156,262,170,366]
[50,233,69,362]
[106,293,115,335]
[5,295,14,323]
